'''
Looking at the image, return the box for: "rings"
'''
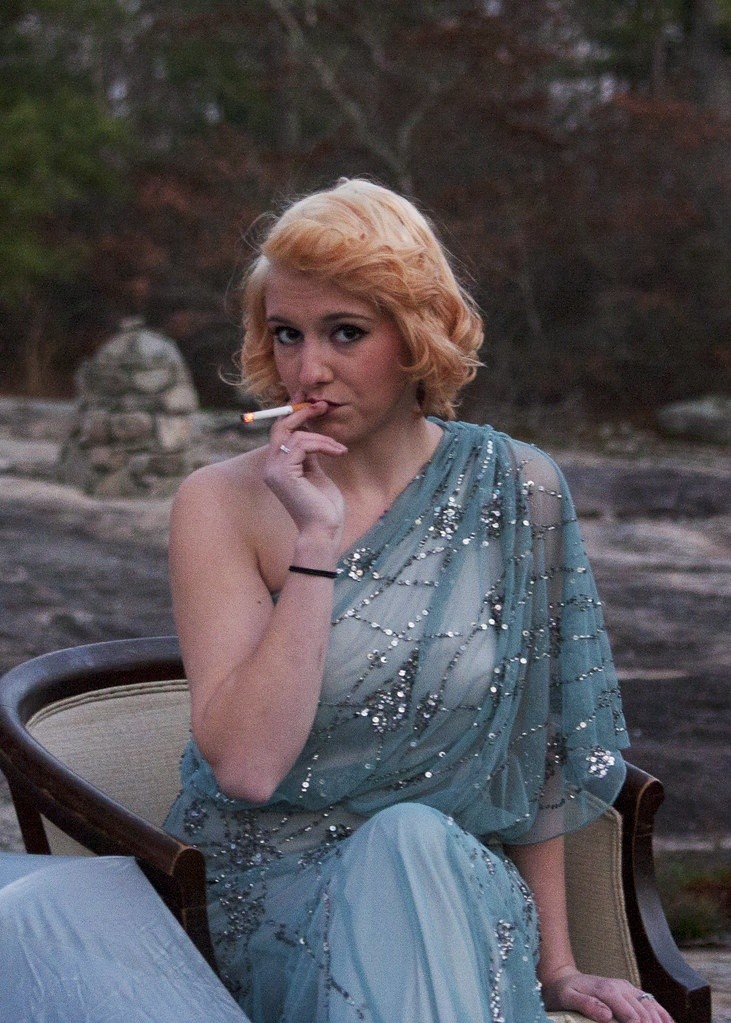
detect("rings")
[639,993,654,1001]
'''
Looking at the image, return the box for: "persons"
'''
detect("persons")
[165,179,676,1023]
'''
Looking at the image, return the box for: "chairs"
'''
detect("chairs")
[0,635,711,1023]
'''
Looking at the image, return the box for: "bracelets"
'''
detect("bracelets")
[286,565,339,580]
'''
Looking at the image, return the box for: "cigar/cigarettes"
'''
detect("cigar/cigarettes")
[241,398,313,425]
